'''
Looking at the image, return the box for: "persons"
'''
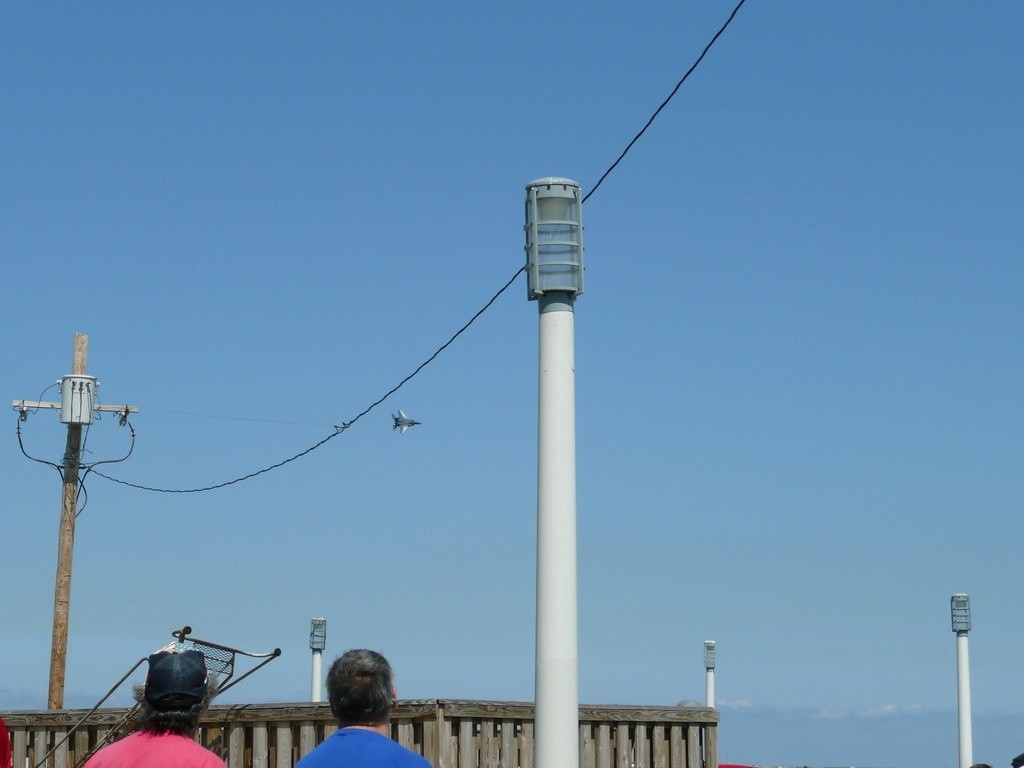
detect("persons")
[83,651,229,768]
[291,649,431,768]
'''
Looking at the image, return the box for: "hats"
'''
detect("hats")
[144,649,209,707]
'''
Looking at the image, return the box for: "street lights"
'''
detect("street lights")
[702,640,717,711]
[309,617,327,704]
[950,592,975,768]
[521,177,587,768]
